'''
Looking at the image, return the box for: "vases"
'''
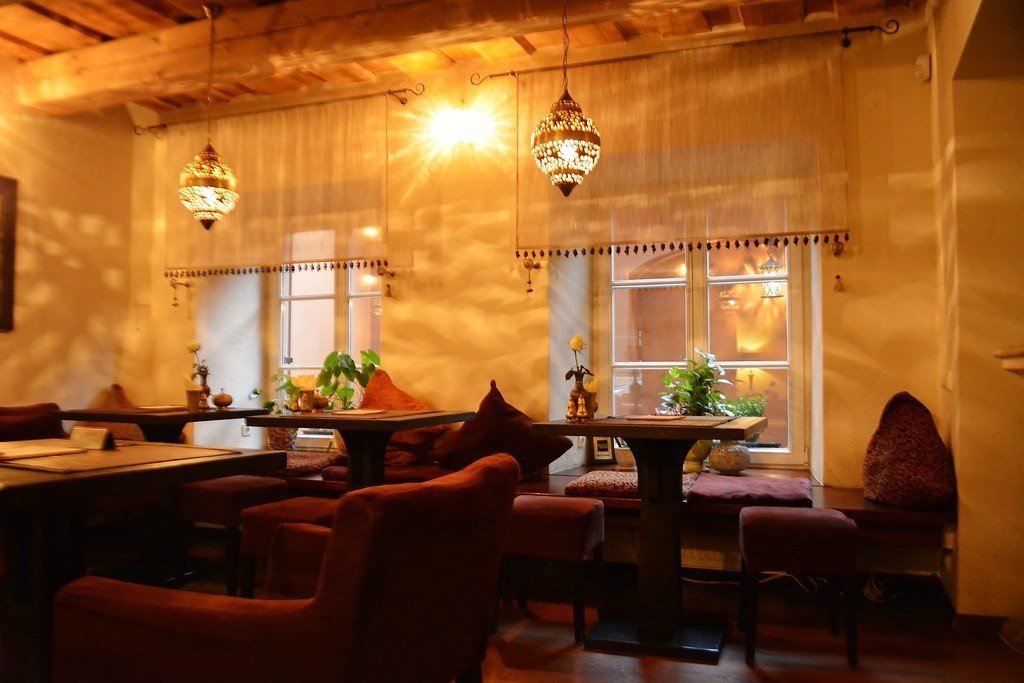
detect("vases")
[199,372,210,409]
[570,375,593,420]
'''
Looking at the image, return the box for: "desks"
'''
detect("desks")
[53,405,268,443]
[534,415,768,660]
[245,408,475,491]
[0,439,287,682]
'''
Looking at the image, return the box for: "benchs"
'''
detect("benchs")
[234,448,956,575]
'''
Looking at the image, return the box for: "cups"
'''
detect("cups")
[615,448,635,469]
[185,390,201,412]
[298,389,314,413]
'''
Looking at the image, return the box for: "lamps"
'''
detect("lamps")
[531,0,600,196]
[759,247,785,297]
[179,3,238,229]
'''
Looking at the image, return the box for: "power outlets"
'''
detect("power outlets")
[242,424,251,436]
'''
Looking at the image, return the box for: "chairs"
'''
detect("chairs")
[49,451,520,682]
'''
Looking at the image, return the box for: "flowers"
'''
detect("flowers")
[185,340,208,381]
[565,335,594,380]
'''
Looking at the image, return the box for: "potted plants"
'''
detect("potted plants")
[253,347,380,453]
[654,348,768,475]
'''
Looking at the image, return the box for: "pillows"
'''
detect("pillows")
[862,391,956,506]
[91,383,147,441]
[428,379,573,485]
[356,369,453,467]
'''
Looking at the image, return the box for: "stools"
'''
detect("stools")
[178,475,338,594]
[509,493,604,642]
[734,507,856,671]
